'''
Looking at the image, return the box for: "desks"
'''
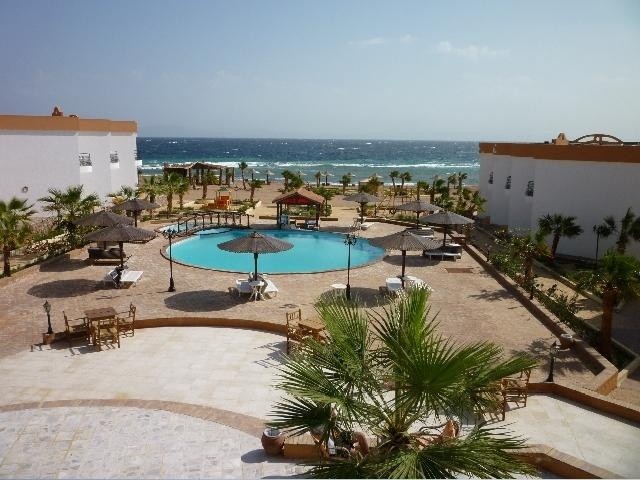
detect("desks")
[343,230,357,298]
[163,227,177,292]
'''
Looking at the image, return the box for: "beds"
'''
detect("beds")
[72,209,134,249]
[85,221,155,268]
[342,190,384,223]
[217,230,294,282]
[392,197,442,228]
[368,229,442,287]
[419,210,475,248]
[113,197,162,228]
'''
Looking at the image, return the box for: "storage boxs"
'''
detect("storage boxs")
[236,271,253,297]
[87,247,144,289]
[348,216,374,230]
[420,226,465,261]
[257,273,277,299]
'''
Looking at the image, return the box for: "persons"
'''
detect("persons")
[328,434,337,458]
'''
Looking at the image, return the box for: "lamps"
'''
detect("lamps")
[418,419,460,448]
[115,302,137,337]
[307,426,350,469]
[471,366,531,422]
[286,308,314,354]
[62,310,90,345]
[90,315,120,351]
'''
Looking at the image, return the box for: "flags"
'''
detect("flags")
[560,333,572,347]
[260,426,285,457]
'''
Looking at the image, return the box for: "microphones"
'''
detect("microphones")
[282,428,319,459]
